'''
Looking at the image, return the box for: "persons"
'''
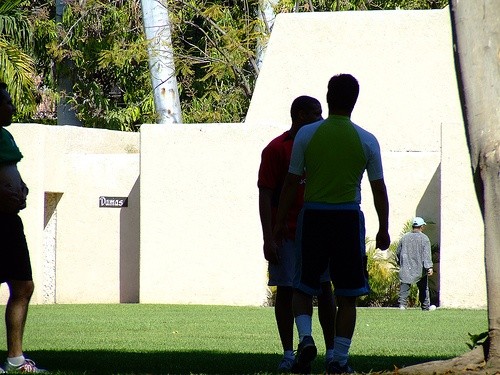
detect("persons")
[0,82,50,375]
[396,217,436,311]
[258,97,335,372]
[272,75,390,373]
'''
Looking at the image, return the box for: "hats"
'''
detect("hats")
[411,217,426,227]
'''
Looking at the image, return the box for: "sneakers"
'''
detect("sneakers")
[278,351,295,372]
[428,305,436,311]
[6,359,49,375]
[325,358,353,374]
[291,336,317,374]
[0,368,5,373]
[400,306,407,309]
